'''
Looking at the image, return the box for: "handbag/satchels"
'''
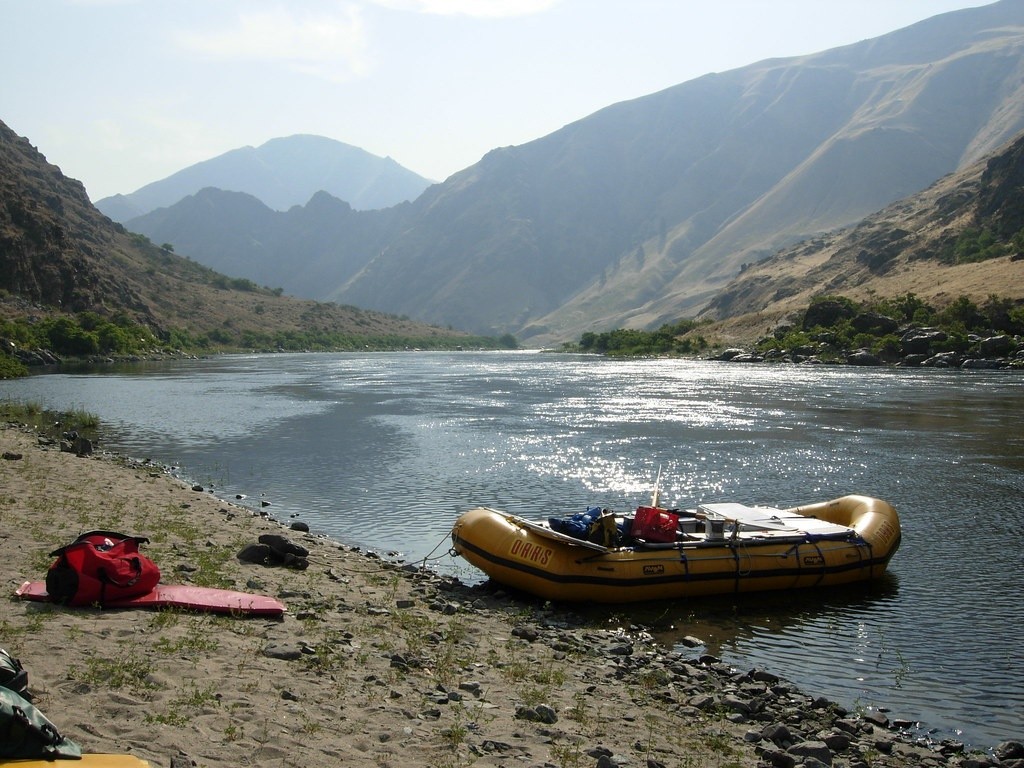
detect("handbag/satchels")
[46,531,160,610]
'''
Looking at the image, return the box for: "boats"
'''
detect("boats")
[450,493,902,606]
